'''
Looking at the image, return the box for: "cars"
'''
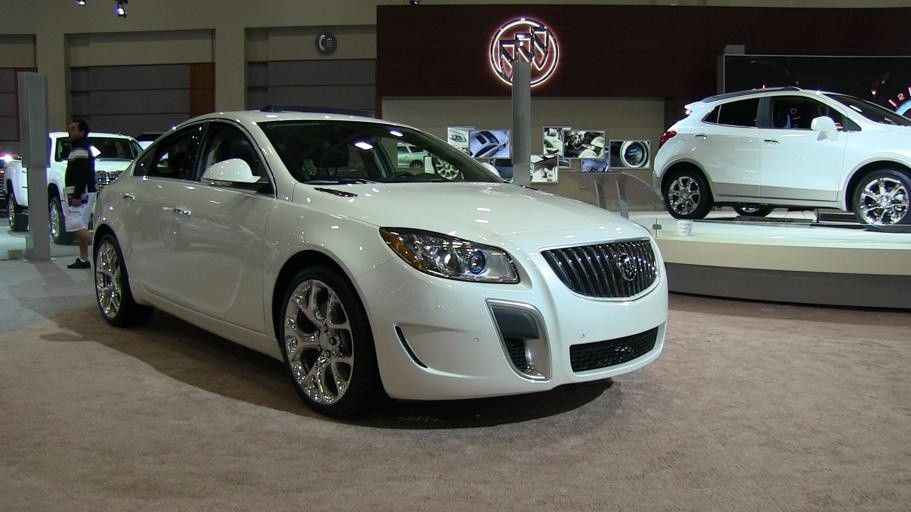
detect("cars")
[3,130,146,245]
[469,129,509,158]
[90,103,671,424]
[649,83,911,234]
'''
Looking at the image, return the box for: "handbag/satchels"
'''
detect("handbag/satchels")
[64,205,86,232]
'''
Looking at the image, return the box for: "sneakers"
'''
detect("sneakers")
[67,258,91,269]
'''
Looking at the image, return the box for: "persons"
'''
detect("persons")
[58,118,98,268]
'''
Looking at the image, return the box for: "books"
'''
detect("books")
[61,184,90,207]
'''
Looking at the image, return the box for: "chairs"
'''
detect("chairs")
[311,139,349,179]
[215,138,259,175]
